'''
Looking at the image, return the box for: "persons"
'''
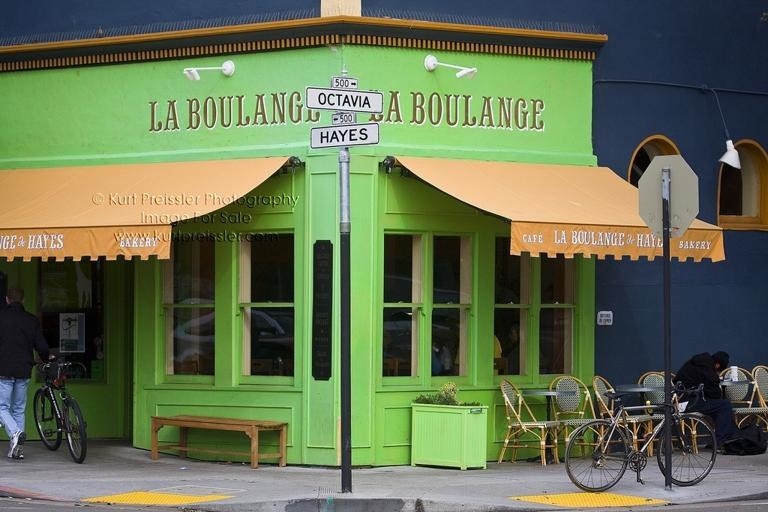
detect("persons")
[672,350,732,452]
[384,313,521,376]
[0,286,49,459]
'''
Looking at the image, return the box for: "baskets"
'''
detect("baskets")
[36,356,65,383]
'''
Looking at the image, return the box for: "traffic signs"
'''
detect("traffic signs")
[310,114,380,149]
[305,76,384,114]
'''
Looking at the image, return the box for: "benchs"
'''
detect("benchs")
[151,414,288,469]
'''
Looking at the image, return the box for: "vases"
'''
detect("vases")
[410,404,488,471]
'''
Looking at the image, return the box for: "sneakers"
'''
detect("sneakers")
[8,431,26,459]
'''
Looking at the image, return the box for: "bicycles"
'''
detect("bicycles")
[564,381,719,493]
[33,356,88,464]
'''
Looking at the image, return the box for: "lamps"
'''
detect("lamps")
[424,54,477,81]
[183,60,235,80]
[701,84,742,171]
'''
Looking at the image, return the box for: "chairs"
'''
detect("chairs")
[497,365,768,464]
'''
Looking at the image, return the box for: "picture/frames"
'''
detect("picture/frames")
[38,257,97,315]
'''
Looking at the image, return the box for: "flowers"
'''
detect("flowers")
[410,381,485,406]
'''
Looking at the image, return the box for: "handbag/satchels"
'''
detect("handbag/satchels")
[722,426,767,455]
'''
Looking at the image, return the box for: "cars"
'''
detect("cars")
[173,297,286,376]
